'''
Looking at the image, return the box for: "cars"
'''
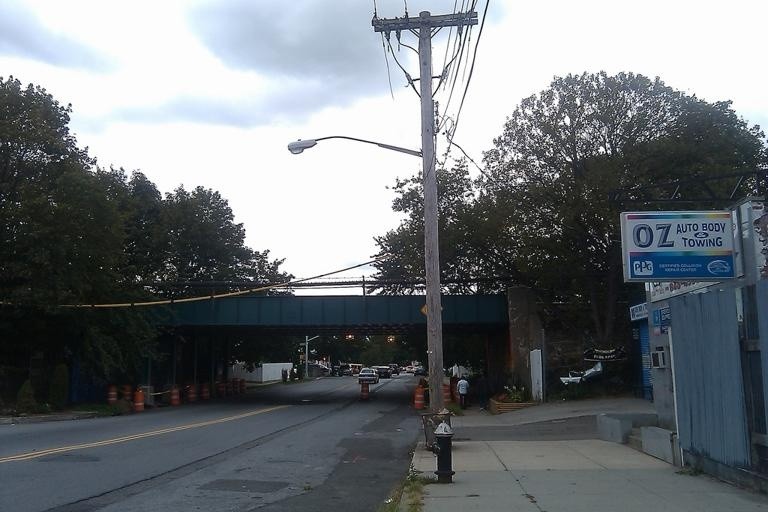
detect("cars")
[308,363,428,384]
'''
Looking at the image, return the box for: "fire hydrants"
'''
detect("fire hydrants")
[424,417,457,482]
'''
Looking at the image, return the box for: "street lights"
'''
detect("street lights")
[288,135,449,450]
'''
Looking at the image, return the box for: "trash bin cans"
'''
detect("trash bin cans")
[323,369,330,376]
[282,368,288,384]
[564,374,604,399]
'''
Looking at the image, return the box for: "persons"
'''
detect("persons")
[456,376,471,410]
[476,377,492,412]
